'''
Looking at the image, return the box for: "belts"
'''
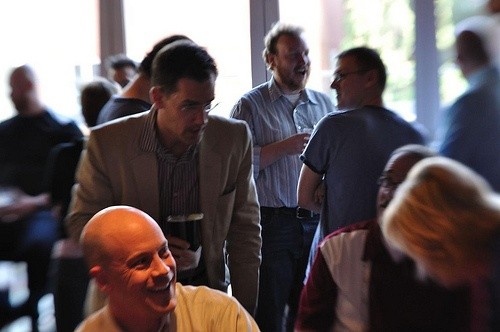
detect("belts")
[260,206,320,219]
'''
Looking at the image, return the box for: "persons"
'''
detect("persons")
[294,144,500,332]
[231,22,339,332]
[73,205,261,332]
[0,36,261,319]
[436,0,500,198]
[296,45,427,286]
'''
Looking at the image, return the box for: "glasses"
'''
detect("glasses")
[377,176,402,188]
[159,87,219,112]
[336,70,363,80]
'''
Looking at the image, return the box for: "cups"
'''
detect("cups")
[166,216,201,252]
[301,128,313,154]
